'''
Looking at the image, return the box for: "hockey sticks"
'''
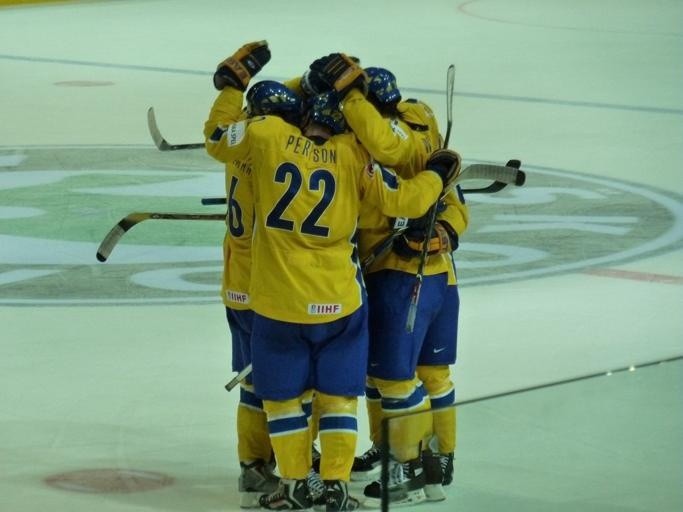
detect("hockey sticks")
[202,159,521,206]
[406,64,457,333]
[201,158,525,391]
[96,211,225,263]
[225,163,524,393]
[147,106,206,150]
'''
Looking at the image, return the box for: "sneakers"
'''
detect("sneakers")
[363,448,454,500]
[349,442,379,472]
[236,462,282,493]
[311,445,323,474]
[323,479,361,512]
[259,476,314,511]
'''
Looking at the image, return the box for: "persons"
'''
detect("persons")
[204,33,469,511]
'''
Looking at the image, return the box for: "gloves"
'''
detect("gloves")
[210,39,272,93]
[424,146,462,189]
[308,51,370,99]
[392,220,460,260]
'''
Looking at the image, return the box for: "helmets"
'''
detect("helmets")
[246,79,303,117]
[359,66,403,108]
[306,90,349,134]
